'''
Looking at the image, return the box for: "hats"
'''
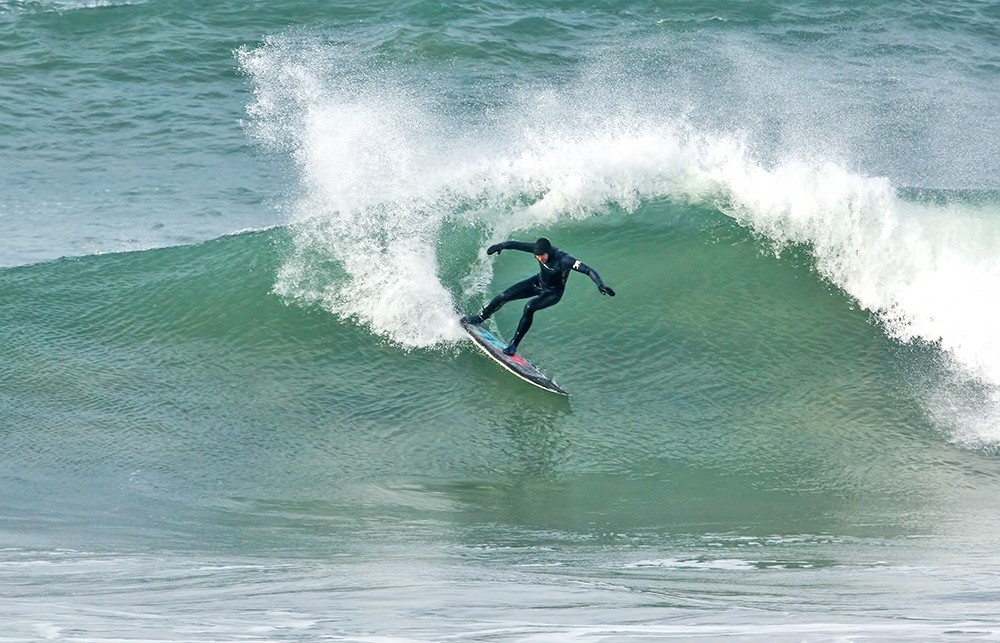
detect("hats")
[534,238,551,262]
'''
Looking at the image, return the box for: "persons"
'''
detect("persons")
[462,238,616,356]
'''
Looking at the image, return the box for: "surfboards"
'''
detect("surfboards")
[461,323,573,397]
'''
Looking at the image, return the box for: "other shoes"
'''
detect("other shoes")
[503,343,517,355]
[462,315,484,324]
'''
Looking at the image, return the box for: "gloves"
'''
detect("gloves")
[598,285,615,297]
[487,245,502,255]
